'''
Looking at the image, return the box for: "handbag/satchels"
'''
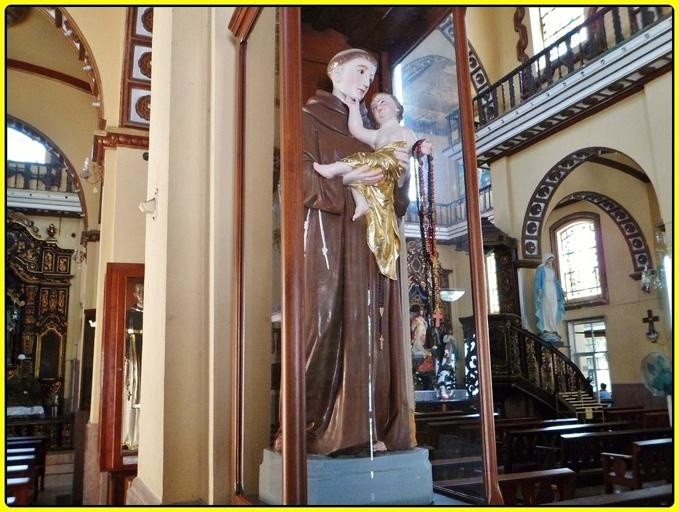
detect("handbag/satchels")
[411,137,420,155]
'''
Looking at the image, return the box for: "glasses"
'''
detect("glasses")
[641,231,668,292]
[80,157,104,193]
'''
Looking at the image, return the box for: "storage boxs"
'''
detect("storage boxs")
[7,436,49,506]
[415,405,673,505]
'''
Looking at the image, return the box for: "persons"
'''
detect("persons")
[443,330,457,356]
[598,382,611,409]
[312,92,418,222]
[122,283,143,450]
[410,307,427,353]
[272,47,417,454]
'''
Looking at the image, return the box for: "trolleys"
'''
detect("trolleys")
[640,353,674,428]
